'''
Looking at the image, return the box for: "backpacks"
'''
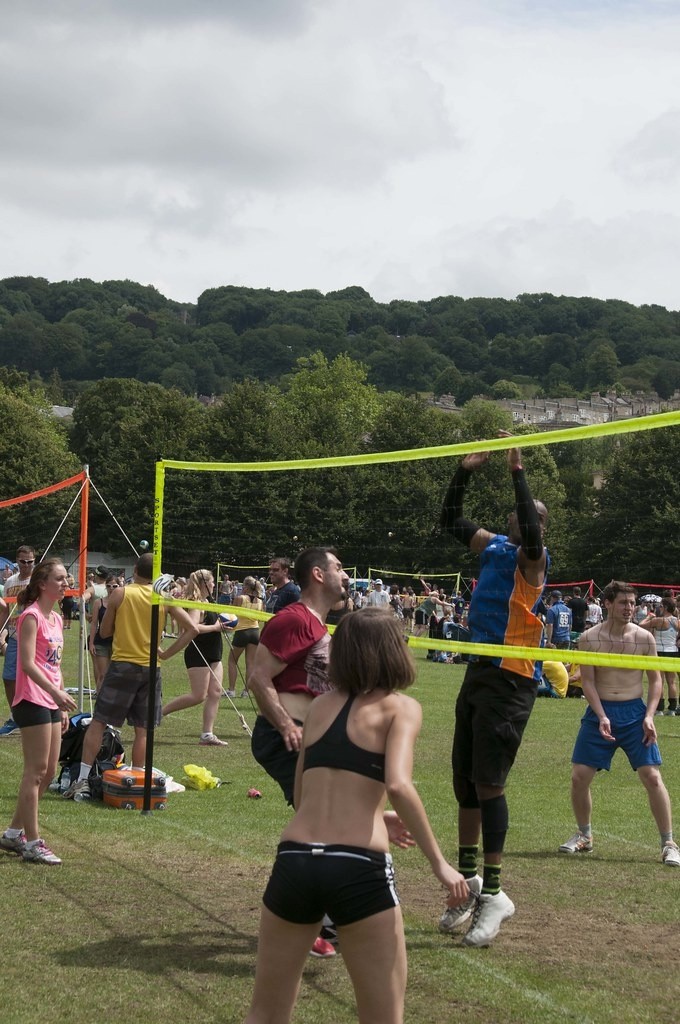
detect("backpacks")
[58,713,126,797]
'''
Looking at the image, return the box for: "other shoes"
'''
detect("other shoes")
[199,735,228,746]
[309,937,336,958]
[63,780,90,798]
[221,689,236,698]
[654,710,676,716]
[241,689,249,699]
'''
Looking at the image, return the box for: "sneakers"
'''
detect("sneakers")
[461,888,515,947]
[559,833,593,853]
[661,841,680,866]
[23,839,61,864]
[0,831,27,855]
[0,718,17,737]
[439,874,484,931]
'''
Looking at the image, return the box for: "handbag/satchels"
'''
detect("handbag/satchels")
[88,762,117,801]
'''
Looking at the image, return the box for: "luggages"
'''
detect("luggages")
[103,770,166,810]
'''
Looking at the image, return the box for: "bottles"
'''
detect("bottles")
[60,767,71,795]
[74,793,91,802]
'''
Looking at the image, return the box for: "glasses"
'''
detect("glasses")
[106,584,119,588]
[269,568,279,571]
[20,558,34,564]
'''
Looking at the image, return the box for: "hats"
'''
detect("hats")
[375,579,382,584]
[551,590,562,598]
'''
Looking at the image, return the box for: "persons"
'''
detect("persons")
[60,552,200,800]
[243,606,471,1024]
[439,429,550,948]
[557,580,680,867]
[249,545,350,958]
[162,570,235,746]
[0,557,78,865]
[0,544,680,741]
